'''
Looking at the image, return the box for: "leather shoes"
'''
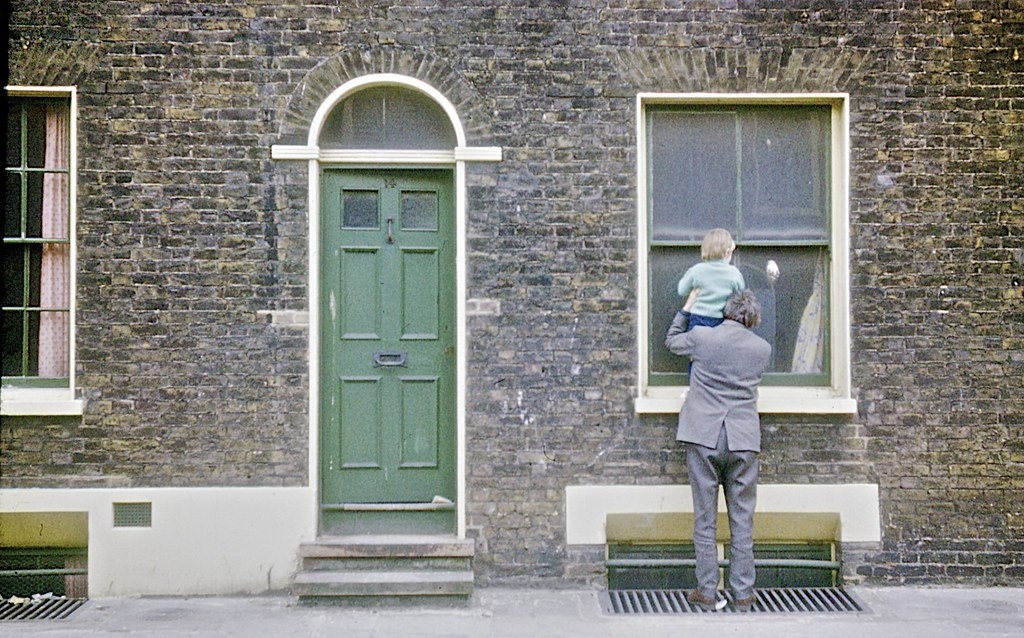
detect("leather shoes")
[736,593,757,610]
[688,589,718,611]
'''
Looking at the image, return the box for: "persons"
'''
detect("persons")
[678,228,745,379]
[664,287,772,610]
